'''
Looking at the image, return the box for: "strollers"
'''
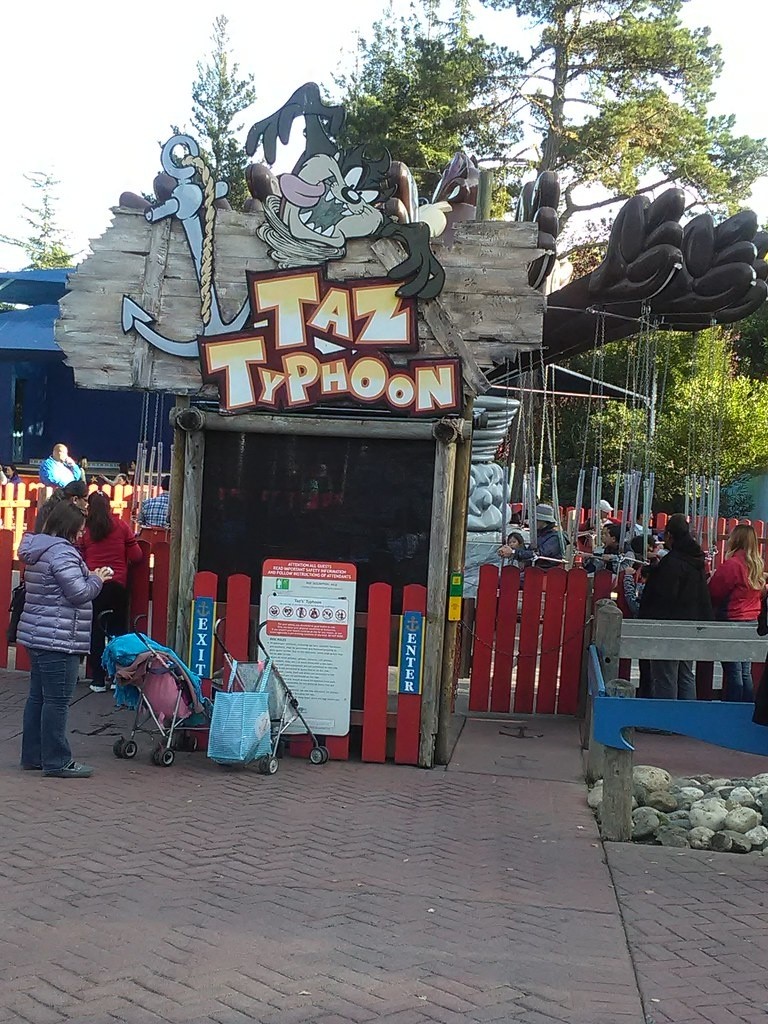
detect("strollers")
[97,611,213,767]
[212,616,330,775]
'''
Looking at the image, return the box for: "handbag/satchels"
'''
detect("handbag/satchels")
[207,657,272,764]
[7,566,25,642]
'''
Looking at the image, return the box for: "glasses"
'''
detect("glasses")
[80,530,86,536]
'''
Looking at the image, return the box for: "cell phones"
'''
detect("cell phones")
[103,567,114,577]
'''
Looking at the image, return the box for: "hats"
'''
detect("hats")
[596,500,614,513]
[536,504,556,523]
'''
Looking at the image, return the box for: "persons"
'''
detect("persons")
[16,500,115,777]
[41,480,89,680]
[752,586,768,726]
[39,440,140,487]
[0,464,22,485]
[497,499,670,621]
[709,524,767,702]
[71,490,143,693]
[639,513,714,736]
[140,475,170,527]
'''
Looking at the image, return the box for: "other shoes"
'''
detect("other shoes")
[635,726,671,735]
[23,764,43,770]
[42,762,93,778]
[110,683,117,690]
[89,681,106,692]
